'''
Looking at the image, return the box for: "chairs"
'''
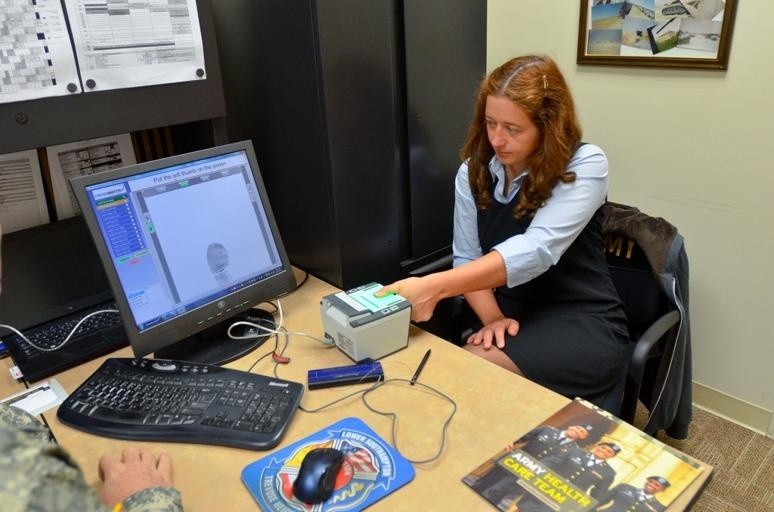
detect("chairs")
[398,196,695,440]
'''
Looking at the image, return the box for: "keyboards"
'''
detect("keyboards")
[56,357,304,451]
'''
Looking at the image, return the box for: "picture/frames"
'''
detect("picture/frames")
[574,0,739,70]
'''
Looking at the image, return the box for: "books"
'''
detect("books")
[460,393,706,511]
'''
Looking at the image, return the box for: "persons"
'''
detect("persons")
[373,53,634,414]
[0,400,183,512]
[472,419,669,510]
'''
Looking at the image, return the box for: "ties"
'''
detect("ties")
[558,438,566,443]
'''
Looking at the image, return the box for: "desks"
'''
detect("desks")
[2,257,715,510]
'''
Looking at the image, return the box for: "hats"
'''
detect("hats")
[647,477,670,489]
[599,443,620,454]
[579,424,593,436]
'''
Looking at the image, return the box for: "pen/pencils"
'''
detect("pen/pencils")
[412,349,431,386]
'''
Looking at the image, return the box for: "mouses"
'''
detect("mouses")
[292,448,343,503]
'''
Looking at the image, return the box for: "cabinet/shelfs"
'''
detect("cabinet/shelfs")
[205,0,488,298]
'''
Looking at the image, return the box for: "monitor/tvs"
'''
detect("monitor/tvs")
[68,139,297,367]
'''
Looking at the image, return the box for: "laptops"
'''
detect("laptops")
[0,215,130,383]
